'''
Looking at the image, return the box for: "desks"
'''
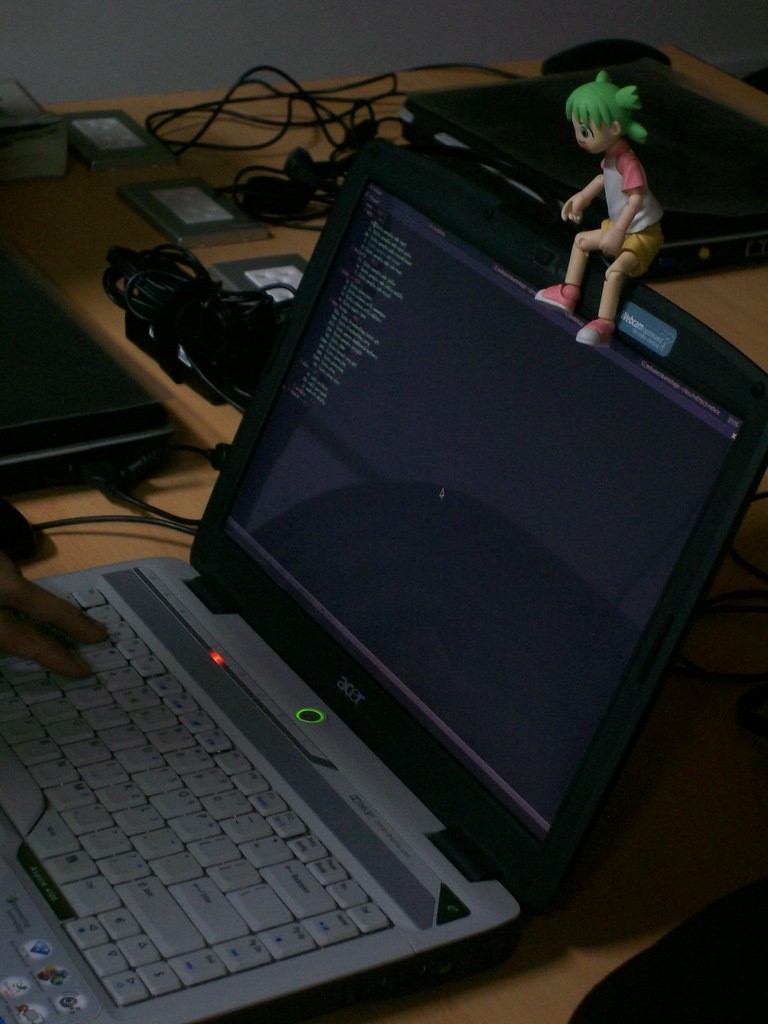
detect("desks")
[1,41,768,1023]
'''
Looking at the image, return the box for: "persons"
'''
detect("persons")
[533,71,662,347]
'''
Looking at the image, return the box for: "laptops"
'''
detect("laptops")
[0,135,768,1023]
[0,256,182,490]
[402,62,768,285]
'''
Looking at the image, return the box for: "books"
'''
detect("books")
[0,79,68,181]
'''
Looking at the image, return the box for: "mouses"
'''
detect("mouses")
[541,39,670,74]
